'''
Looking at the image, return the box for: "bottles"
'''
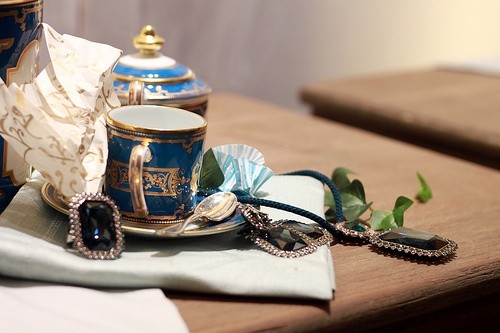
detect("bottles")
[1,0,44,216]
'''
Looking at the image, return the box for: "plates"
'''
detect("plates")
[39,174,262,236]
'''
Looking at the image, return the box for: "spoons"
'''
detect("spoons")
[153,191,237,239]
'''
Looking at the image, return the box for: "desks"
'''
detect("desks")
[0,86,500,333]
[299,64,500,174]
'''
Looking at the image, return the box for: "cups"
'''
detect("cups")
[103,104,209,227]
[106,21,211,120]
